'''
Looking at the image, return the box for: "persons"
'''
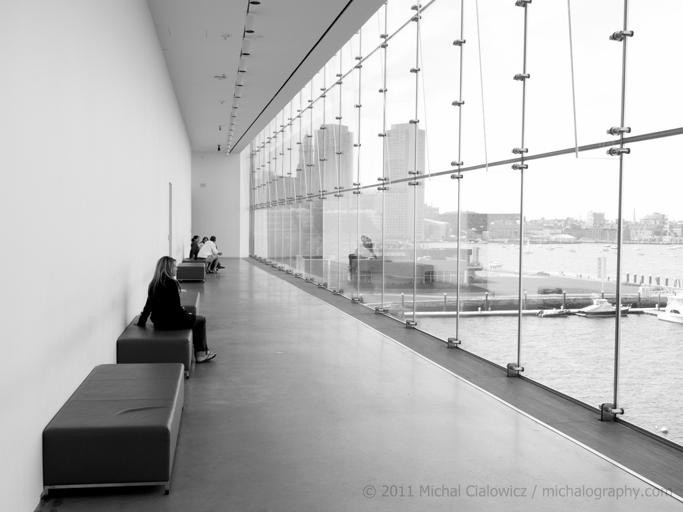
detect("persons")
[134,255,217,364]
[348,235,376,273]
[186,235,225,274]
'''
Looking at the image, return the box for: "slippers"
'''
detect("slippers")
[196,351,216,364]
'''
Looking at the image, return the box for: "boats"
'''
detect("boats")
[578,299,629,315]
[537,309,570,317]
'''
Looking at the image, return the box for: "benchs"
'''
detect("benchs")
[352,259,391,273]
[117,314,194,379]
[177,263,205,283]
[384,262,434,285]
[42,362,185,503]
[177,291,201,317]
[182,258,207,273]
